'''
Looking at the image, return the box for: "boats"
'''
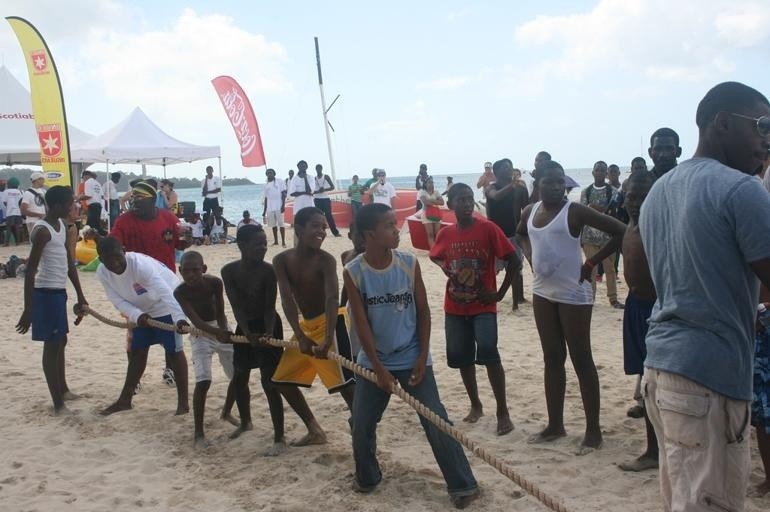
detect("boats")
[270,188,431,226]
[407,205,487,252]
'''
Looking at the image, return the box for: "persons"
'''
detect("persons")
[15,185,89,413]
[1,173,48,245]
[95,236,191,415]
[430,182,522,435]
[478,151,628,454]
[221,224,286,455]
[582,128,682,471]
[273,208,368,446]
[237,210,258,229]
[348,168,396,206]
[188,165,237,243]
[150,179,177,214]
[172,252,240,454]
[637,81,770,511]
[262,161,341,247]
[109,182,186,396]
[78,170,141,249]
[416,164,454,248]
[343,204,480,507]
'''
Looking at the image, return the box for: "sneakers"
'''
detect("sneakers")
[134,382,142,394]
[161,368,176,388]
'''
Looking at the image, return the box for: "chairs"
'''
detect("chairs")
[176,201,204,226]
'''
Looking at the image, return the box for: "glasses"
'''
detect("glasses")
[728,111,770,138]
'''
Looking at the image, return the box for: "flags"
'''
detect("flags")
[6,16,71,188]
[211,75,266,167]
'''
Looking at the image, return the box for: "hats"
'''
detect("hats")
[375,168,386,176]
[419,163,427,169]
[484,161,492,168]
[29,171,48,183]
[8,177,20,187]
[134,179,157,198]
